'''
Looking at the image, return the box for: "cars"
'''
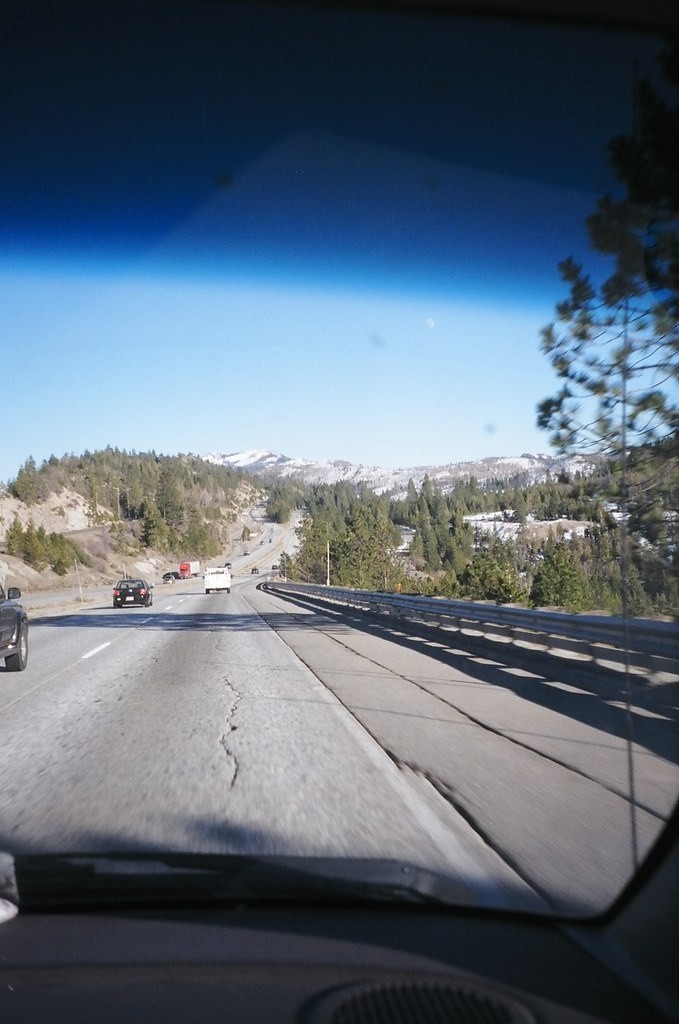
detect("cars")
[112,578,154,608]
[0,584,29,672]
[162,574,176,585]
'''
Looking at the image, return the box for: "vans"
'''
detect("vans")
[202,567,233,594]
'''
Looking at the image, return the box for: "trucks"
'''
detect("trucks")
[180,561,200,579]
